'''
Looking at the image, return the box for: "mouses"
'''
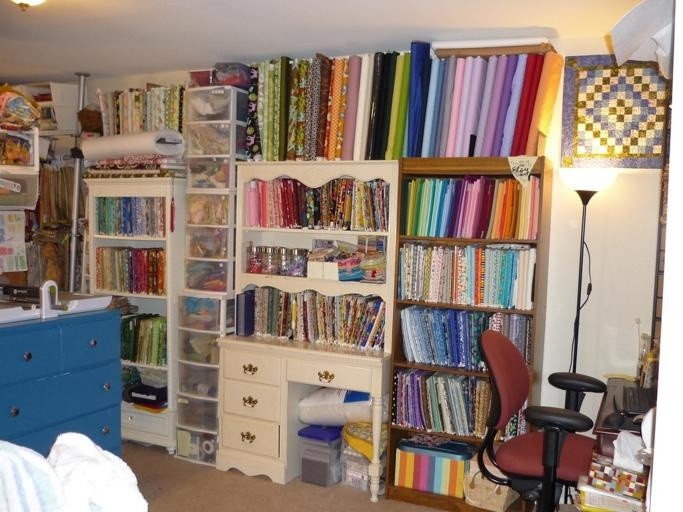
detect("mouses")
[632,415,644,424]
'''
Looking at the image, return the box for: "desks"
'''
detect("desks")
[592,378,654,460]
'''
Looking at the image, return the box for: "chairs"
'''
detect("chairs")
[478,330,607,512]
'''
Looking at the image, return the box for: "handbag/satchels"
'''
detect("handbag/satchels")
[464,473,522,512]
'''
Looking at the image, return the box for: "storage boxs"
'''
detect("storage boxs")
[172,84,251,467]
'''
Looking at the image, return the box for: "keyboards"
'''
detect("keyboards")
[614,384,650,415]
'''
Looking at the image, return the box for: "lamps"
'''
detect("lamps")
[557,168,624,373]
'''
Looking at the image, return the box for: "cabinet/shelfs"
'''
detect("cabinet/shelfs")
[81,178,185,457]
[0,293,122,460]
[384,155,553,511]
[215,158,400,503]
